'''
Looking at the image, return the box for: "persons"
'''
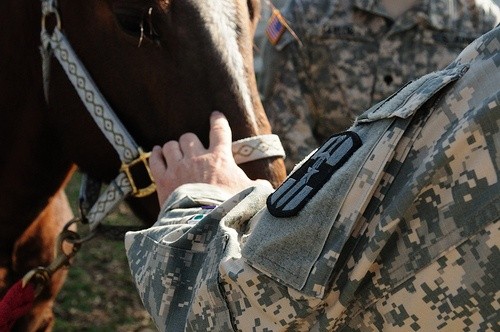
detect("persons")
[255,0,500,175]
[125,23,500,332]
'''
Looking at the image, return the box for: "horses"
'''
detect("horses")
[0,0,287,331]
